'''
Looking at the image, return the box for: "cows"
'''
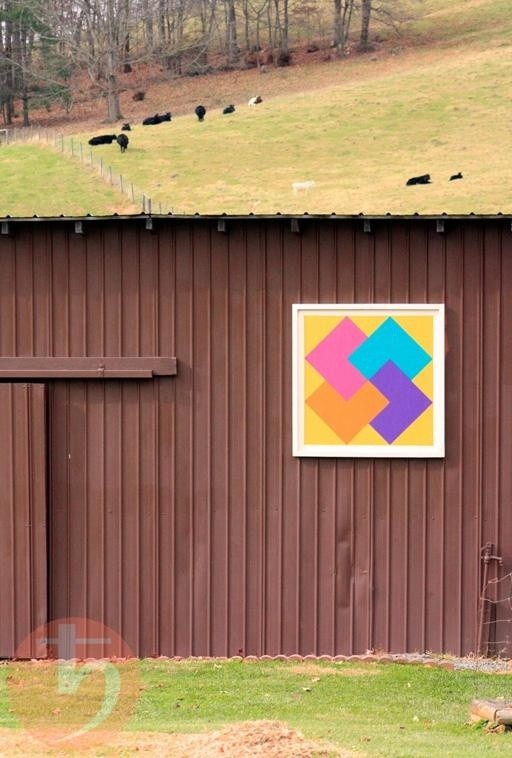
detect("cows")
[121,122,131,132]
[406,173,431,186]
[88,134,116,146]
[293,180,317,195]
[223,104,235,115]
[247,95,263,106]
[142,111,172,126]
[449,172,463,180]
[117,134,128,153]
[195,105,206,121]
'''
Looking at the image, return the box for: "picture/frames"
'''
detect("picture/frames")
[292,303,446,459]
